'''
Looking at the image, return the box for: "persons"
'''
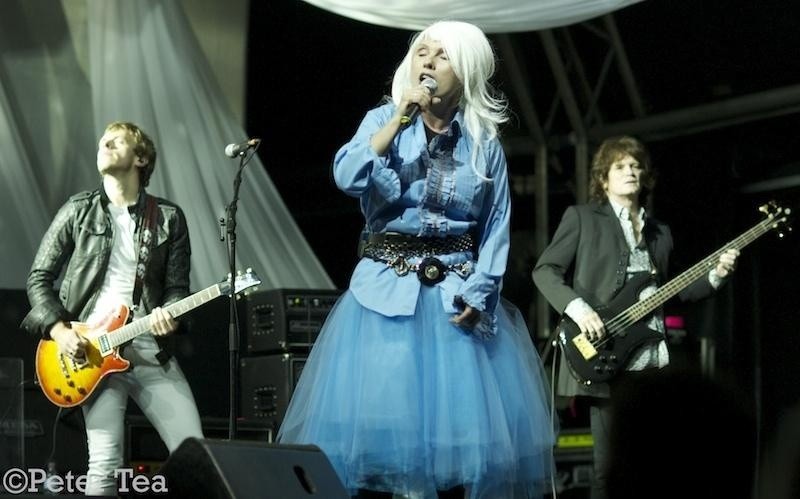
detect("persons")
[276,20,562,499]
[18,122,205,496]
[531,133,741,499]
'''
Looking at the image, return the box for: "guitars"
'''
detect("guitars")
[35,268,262,409]
[553,201,792,386]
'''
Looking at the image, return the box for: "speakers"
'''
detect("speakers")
[147,437,347,499]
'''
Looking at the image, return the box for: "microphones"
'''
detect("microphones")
[225,139,260,158]
[399,78,438,130]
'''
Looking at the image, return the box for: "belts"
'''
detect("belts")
[357,227,476,261]
